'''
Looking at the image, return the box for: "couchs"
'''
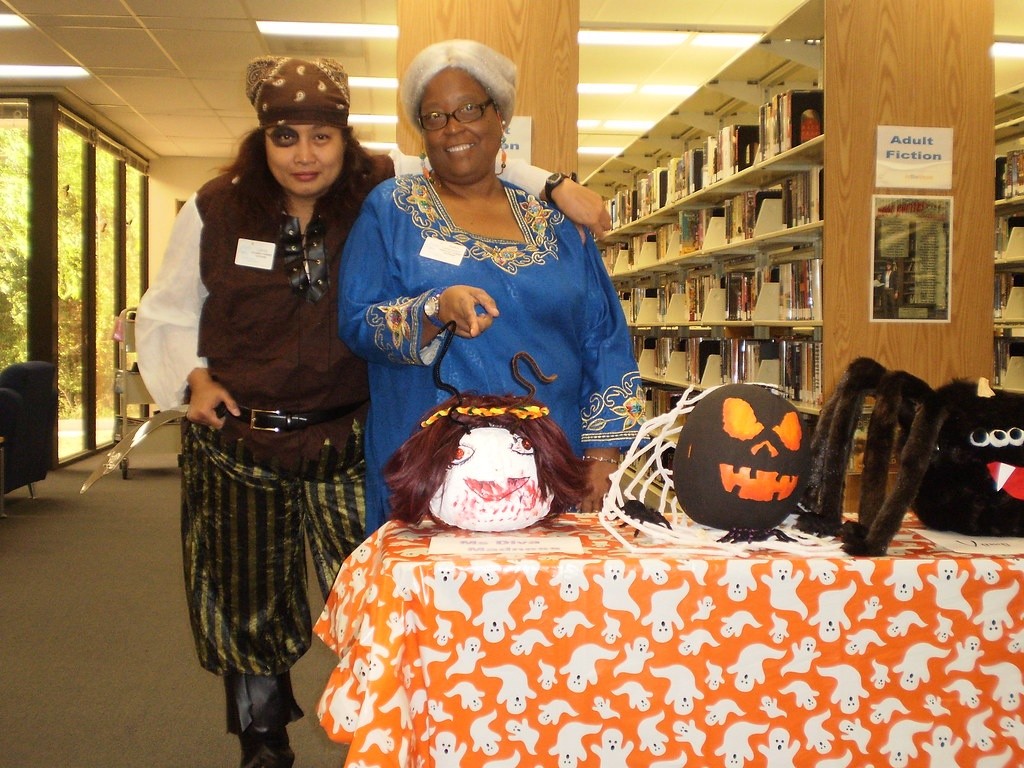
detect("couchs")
[0,363,55,498]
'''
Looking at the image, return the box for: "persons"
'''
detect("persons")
[135,59,612,768]
[338,41,652,540]
[880,259,899,317]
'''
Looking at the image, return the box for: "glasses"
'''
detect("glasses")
[417,99,491,131]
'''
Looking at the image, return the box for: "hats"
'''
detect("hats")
[246,56,352,130]
[401,38,518,136]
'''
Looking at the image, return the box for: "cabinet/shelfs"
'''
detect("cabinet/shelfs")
[578,0,995,513]
[994,82,1024,392]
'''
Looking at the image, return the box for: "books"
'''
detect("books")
[992,150,1024,387]
[594,89,824,490]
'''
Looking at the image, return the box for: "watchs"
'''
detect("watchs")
[423,286,445,328]
[545,172,577,205]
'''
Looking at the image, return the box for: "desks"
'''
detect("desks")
[318,515,1024,768]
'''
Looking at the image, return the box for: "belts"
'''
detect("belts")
[184,386,369,433]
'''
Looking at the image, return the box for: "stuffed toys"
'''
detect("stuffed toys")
[381,321,593,532]
[797,357,1024,556]
[673,383,809,529]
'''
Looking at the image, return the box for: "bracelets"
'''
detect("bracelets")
[582,456,618,465]
